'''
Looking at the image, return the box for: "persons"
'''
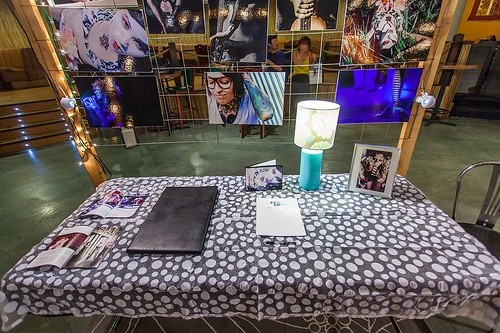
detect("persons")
[147,0,181,35]
[206,72,274,125]
[208,0,268,62]
[363,0,432,62]
[358,150,387,192]
[82,78,134,129]
[247,167,283,190]
[289,0,315,19]
[265,33,290,81]
[57,8,150,73]
[372,70,408,119]
[292,36,319,82]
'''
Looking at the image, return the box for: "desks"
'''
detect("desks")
[0,173,500,333]
[423,62,482,127]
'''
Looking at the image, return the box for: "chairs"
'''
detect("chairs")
[452,160,500,259]
[150,41,341,139]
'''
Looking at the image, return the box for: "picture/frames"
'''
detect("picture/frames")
[347,143,401,199]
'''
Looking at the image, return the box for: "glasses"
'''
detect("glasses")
[206,76,234,90]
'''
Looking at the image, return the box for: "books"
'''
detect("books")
[29,223,123,269]
[127,186,219,256]
[79,189,150,218]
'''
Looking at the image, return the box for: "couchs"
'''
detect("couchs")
[0,48,49,90]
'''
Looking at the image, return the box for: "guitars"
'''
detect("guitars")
[290,15,327,30]
